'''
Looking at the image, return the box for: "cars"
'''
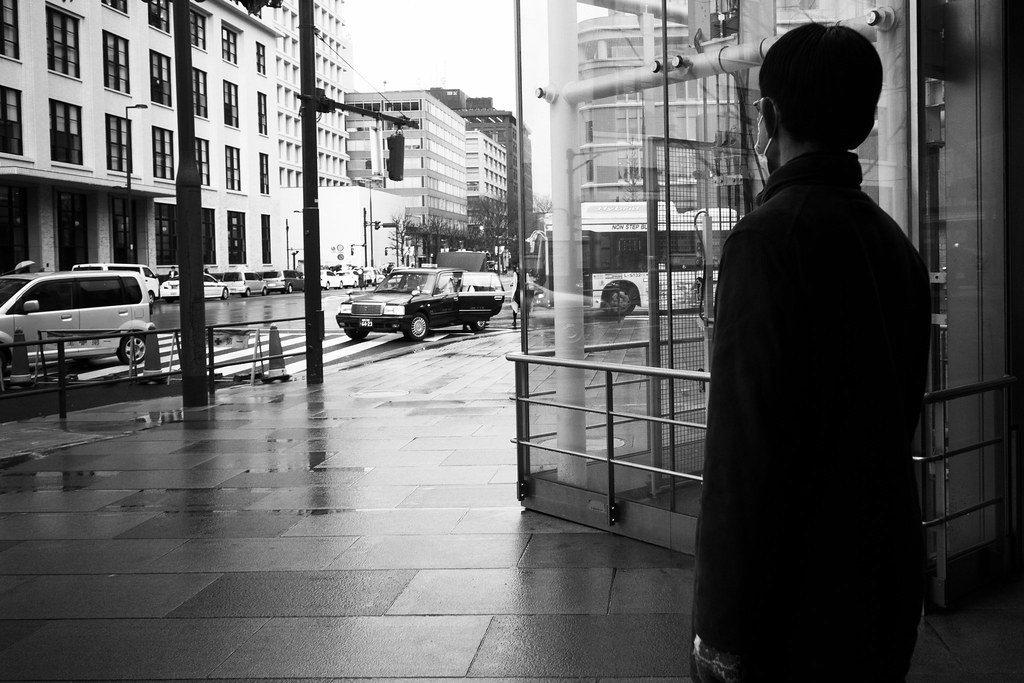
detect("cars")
[335,252,505,341]
[334,271,357,289]
[160,272,229,304]
[320,270,343,290]
[387,267,422,287]
[354,267,376,288]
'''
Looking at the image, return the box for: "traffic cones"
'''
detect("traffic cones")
[9,329,36,389]
[261,323,292,383]
[137,325,168,384]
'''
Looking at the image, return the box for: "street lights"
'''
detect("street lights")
[293,251,299,270]
[353,176,382,267]
[125,104,148,263]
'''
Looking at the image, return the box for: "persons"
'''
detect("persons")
[357,266,365,291]
[168,266,178,280]
[510,265,534,327]
[691,22,930,683]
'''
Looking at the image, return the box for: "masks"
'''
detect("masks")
[753,97,778,185]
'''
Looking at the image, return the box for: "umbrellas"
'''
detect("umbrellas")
[14,261,35,273]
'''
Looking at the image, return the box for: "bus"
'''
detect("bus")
[525,201,746,316]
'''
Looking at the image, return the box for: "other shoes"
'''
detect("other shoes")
[512,322,516,326]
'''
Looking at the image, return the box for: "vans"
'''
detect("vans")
[219,271,268,297]
[262,269,304,294]
[0,270,156,375]
[72,263,160,304]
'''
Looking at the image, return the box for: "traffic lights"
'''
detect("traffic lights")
[351,245,354,255]
[375,221,381,230]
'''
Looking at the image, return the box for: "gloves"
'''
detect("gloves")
[693,633,746,683]
